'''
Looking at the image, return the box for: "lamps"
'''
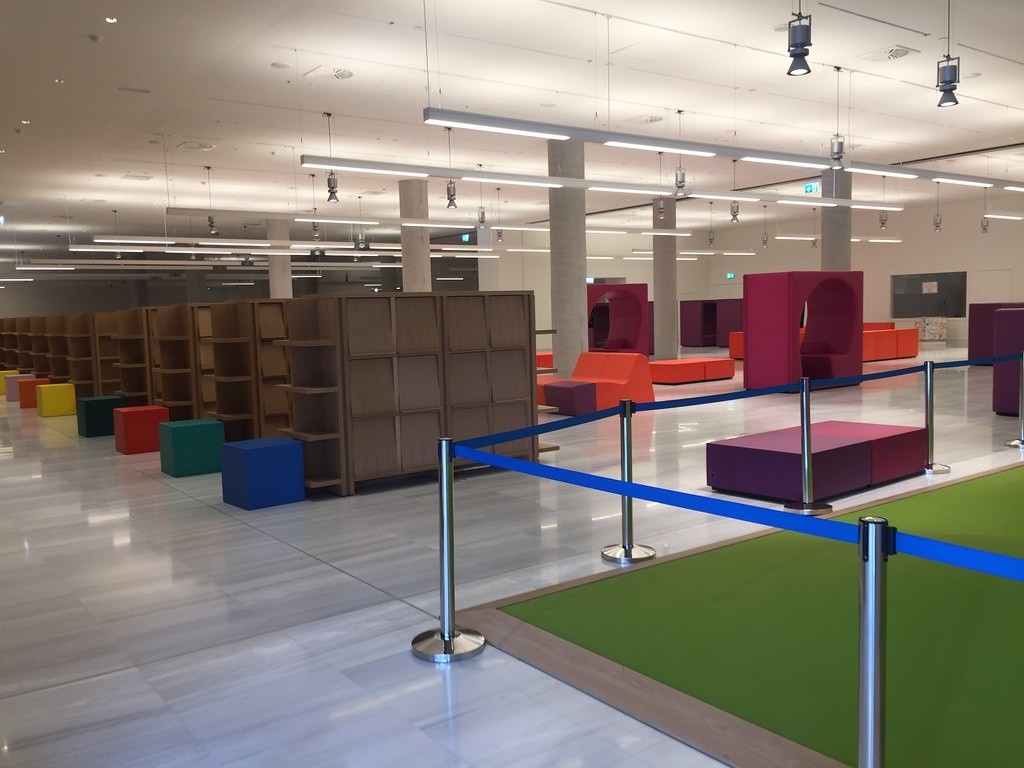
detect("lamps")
[0,0,1024,282]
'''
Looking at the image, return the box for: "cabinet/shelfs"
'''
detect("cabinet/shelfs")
[0,289,537,497]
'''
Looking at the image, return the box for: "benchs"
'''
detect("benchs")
[536,352,655,416]
[589,338,634,353]
